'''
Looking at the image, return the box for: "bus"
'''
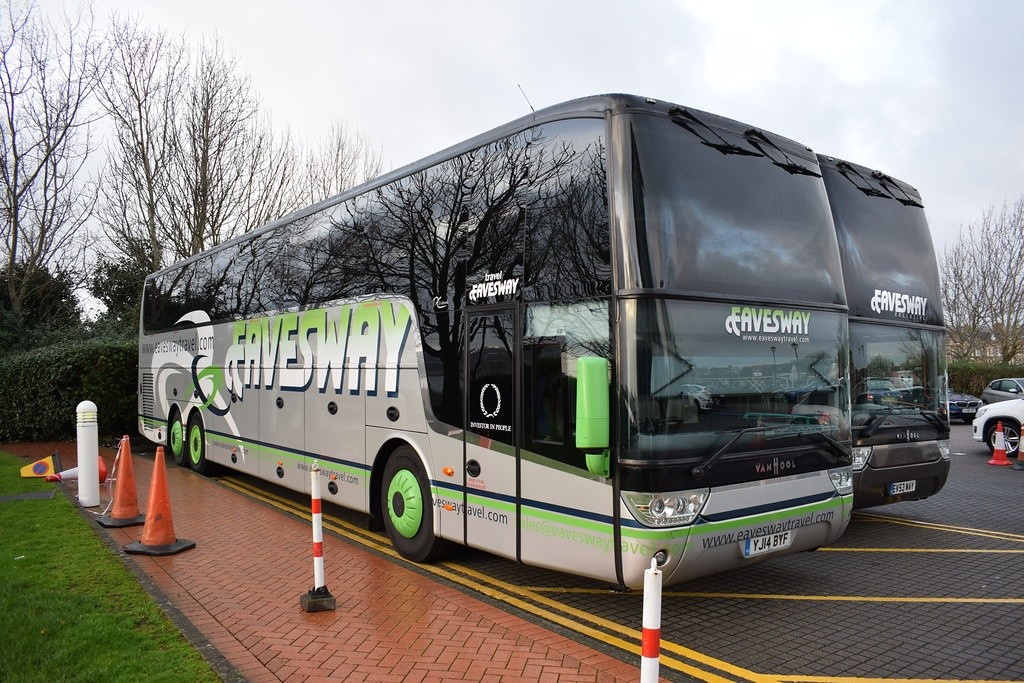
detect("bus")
[137,90,857,592]
[812,147,956,512]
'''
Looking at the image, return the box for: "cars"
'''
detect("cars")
[971,396,1024,457]
[979,377,1024,409]
[945,387,983,426]
[675,375,928,412]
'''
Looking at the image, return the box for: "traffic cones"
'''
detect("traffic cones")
[122,442,201,559]
[20,449,65,478]
[1009,424,1024,471]
[96,433,148,529]
[44,453,108,483]
[985,420,1014,466]
